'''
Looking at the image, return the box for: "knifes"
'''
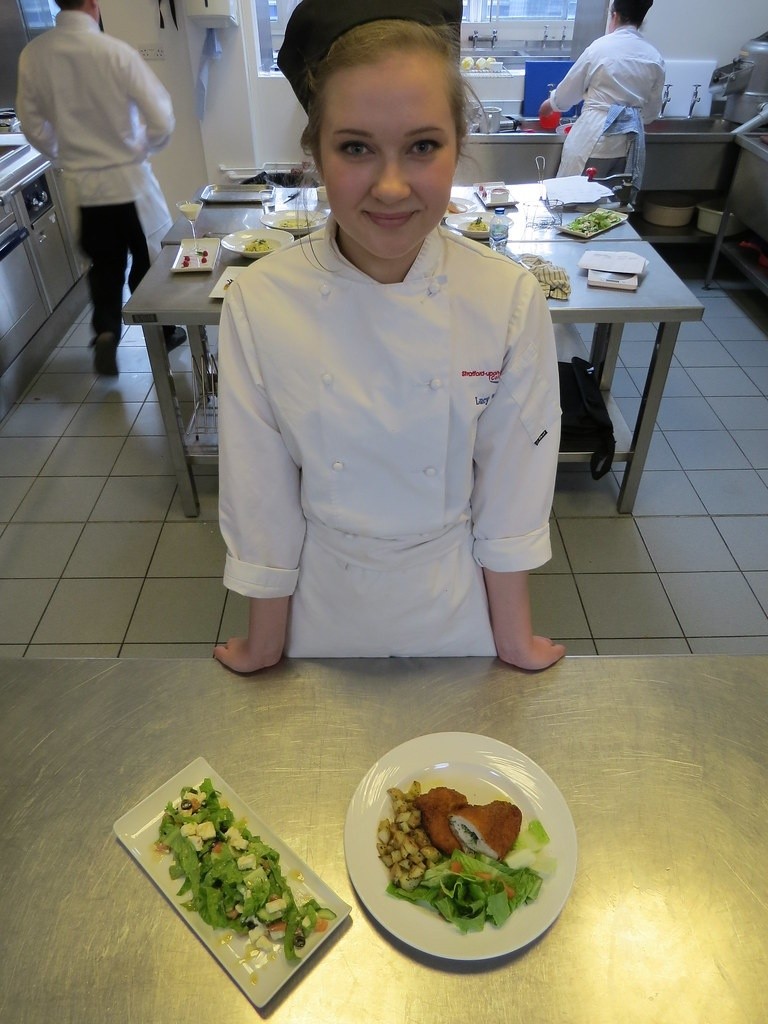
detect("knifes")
[284,190,299,203]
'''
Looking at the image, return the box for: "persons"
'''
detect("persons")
[537,0,667,207]
[211,0,568,673]
[15,0,192,376]
[274,0,462,119]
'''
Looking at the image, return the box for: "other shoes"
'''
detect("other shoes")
[166,326,188,353]
[87,333,120,377]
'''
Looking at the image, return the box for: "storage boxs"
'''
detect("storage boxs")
[696,196,750,237]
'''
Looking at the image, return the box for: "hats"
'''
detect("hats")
[277,0,463,118]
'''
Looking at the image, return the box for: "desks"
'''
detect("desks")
[117,182,704,519]
[0,655,768,1024]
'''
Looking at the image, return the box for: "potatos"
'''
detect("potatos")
[377,780,441,893]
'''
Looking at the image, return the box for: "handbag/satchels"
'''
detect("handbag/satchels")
[558,356,616,479]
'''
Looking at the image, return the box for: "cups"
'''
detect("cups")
[258,189,276,214]
[316,186,328,202]
[479,106,502,132]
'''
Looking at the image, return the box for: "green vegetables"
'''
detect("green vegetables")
[385,849,543,933]
[154,778,336,960]
[568,210,622,234]
[252,239,266,244]
[476,216,482,224]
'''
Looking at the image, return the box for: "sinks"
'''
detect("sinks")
[458,49,523,57]
[526,49,572,57]
[478,118,734,133]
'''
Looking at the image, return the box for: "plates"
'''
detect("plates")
[554,208,629,238]
[208,266,247,298]
[344,730,576,961]
[200,184,276,204]
[170,238,220,272]
[443,197,476,217]
[112,756,351,1008]
[471,181,518,207]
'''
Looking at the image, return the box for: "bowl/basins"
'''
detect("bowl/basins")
[642,196,695,227]
[445,211,514,240]
[259,210,328,235]
[696,200,745,235]
[220,229,294,258]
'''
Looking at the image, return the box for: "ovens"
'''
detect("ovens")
[0,144,75,375]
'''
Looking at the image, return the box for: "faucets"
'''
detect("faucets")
[658,83,673,118]
[543,24,567,51]
[688,84,701,117]
[467,29,498,49]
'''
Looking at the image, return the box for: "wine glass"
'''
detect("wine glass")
[175,200,204,252]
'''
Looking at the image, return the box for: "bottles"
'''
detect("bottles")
[489,208,508,258]
